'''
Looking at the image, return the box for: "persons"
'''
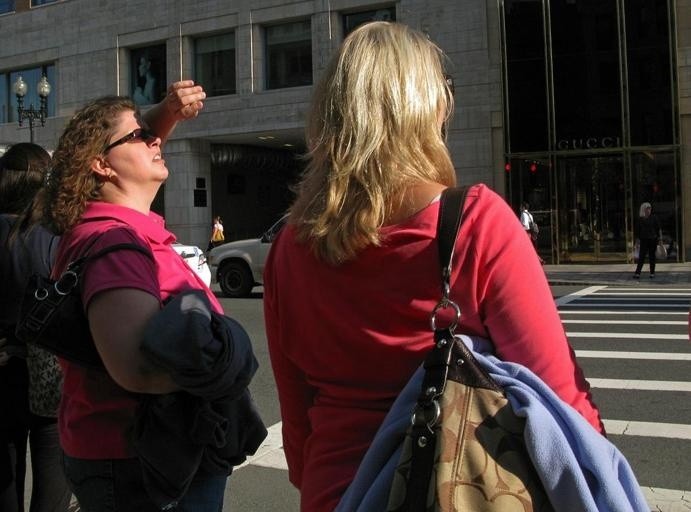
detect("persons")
[261,18,609,512]
[519,200,546,266]
[46,76,261,512]
[210,215,225,248]
[633,201,663,279]
[0,141,72,512]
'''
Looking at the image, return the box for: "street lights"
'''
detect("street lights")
[11,74,51,143]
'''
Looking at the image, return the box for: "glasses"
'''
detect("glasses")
[442,74,455,99]
[103,129,157,155]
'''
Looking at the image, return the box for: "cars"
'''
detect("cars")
[206,212,293,297]
[170,240,211,289]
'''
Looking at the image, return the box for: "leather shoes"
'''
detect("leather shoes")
[650,274,655,279]
[634,275,640,279]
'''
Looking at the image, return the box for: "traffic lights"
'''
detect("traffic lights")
[504,162,510,173]
[529,164,538,172]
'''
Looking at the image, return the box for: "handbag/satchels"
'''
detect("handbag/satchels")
[656,245,667,259]
[387,298,553,507]
[531,222,539,240]
[214,230,224,242]
[24,343,64,417]
[18,277,99,373]
